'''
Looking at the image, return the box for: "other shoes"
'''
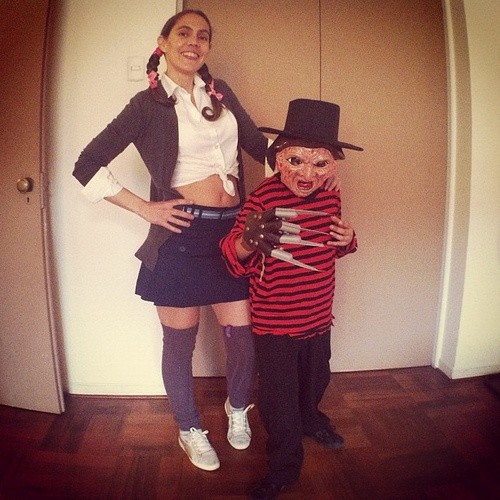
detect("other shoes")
[303,425,345,448]
[248,468,286,500]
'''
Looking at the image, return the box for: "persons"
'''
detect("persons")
[219,98,358,500]
[71,9,345,470]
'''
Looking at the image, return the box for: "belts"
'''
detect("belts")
[182,206,241,219]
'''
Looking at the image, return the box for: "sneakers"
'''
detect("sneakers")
[178,427,220,471]
[224,396,255,449]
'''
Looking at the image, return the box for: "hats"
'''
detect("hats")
[257,99,363,150]
